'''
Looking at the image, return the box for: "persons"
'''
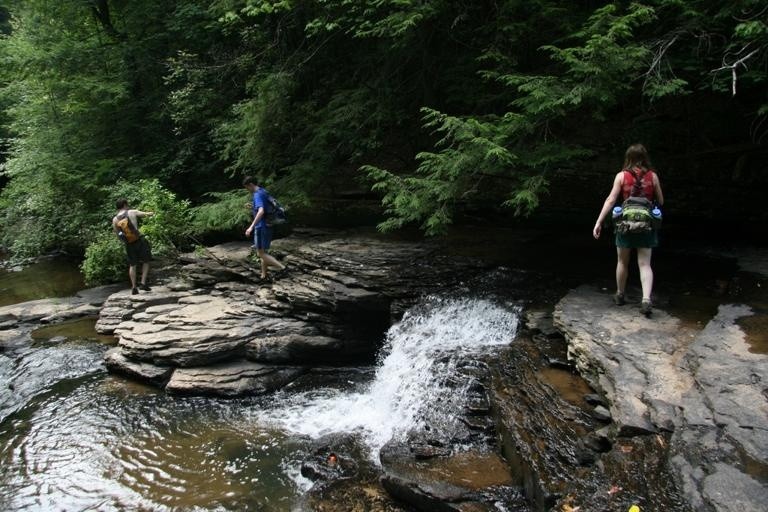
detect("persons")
[242,176,288,282]
[112,200,153,294]
[593,144,664,314]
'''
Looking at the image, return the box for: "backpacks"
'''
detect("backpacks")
[615,168,661,237]
[258,191,286,228]
[116,211,140,243]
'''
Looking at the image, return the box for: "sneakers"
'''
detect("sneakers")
[139,284,152,291]
[132,288,139,295]
[255,275,271,284]
[611,292,626,304]
[275,264,291,278]
[639,302,653,314]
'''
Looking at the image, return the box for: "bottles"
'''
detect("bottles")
[612,205,623,219]
[277,204,285,222]
[117,231,123,238]
[651,206,662,220]
